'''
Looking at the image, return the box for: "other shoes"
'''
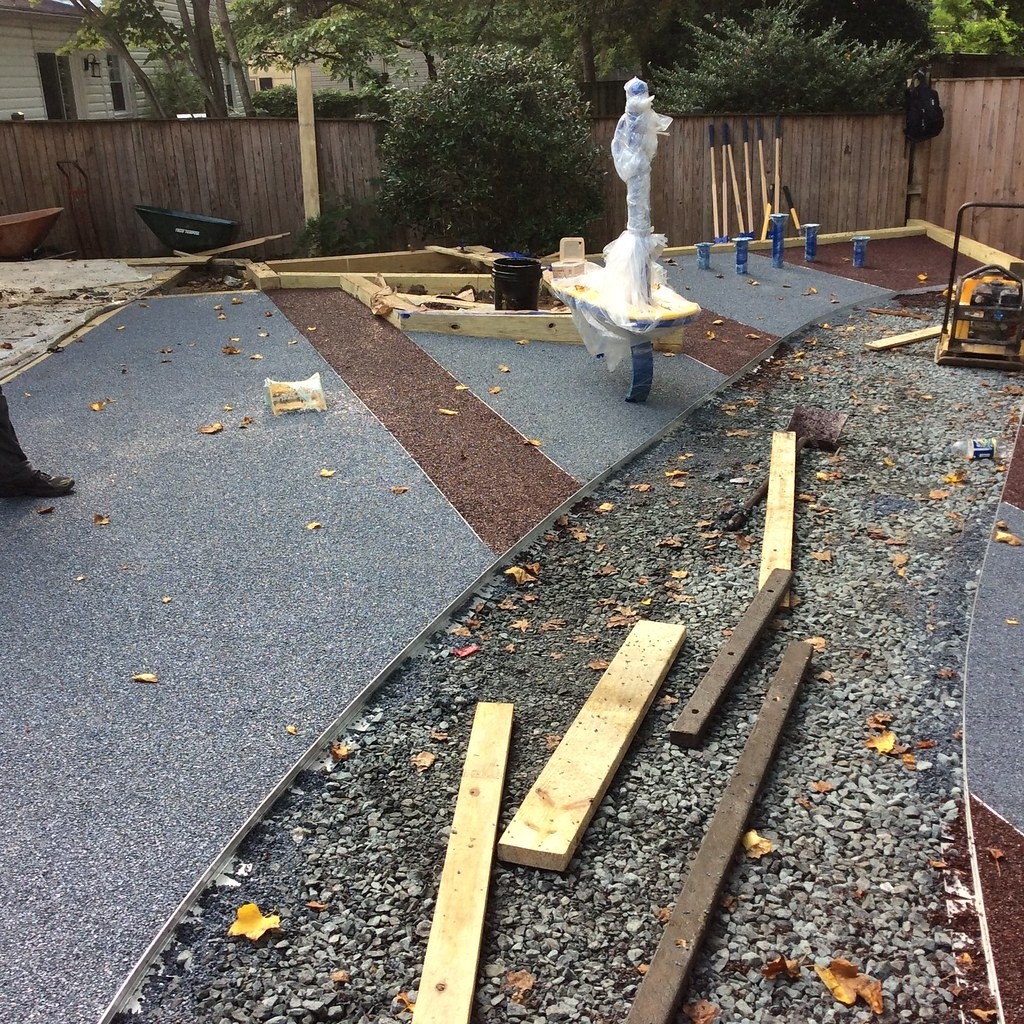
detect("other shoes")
[0,471,75,497]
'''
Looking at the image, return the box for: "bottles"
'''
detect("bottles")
[946,437,1009,459]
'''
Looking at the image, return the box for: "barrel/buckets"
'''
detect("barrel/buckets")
[489,257,544,310]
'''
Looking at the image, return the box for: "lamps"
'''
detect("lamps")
[84,53,102,78]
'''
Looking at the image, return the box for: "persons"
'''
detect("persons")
[0,385,75,497]
